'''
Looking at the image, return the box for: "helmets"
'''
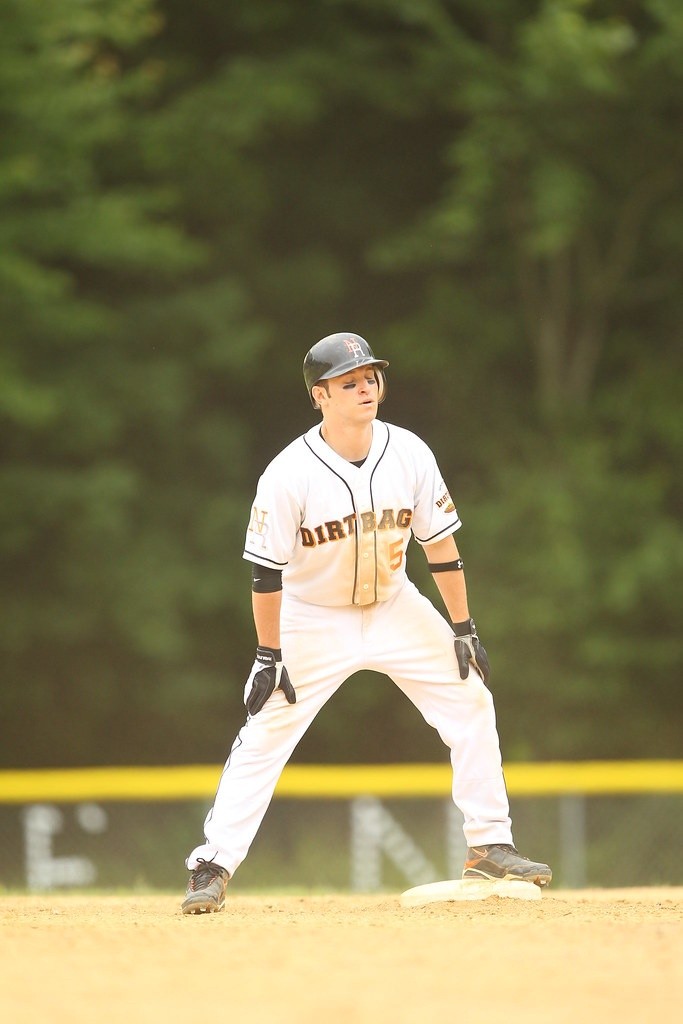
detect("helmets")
[302,332,390,409]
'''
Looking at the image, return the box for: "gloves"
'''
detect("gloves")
[242,646,297,717]
[450,618,491,687]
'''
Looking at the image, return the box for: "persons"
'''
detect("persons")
[182,332,553,914]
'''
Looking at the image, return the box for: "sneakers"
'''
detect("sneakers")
[462,843,552,888]
[181,850,229,914]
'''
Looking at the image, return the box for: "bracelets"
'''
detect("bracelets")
[427,558,465,572]
